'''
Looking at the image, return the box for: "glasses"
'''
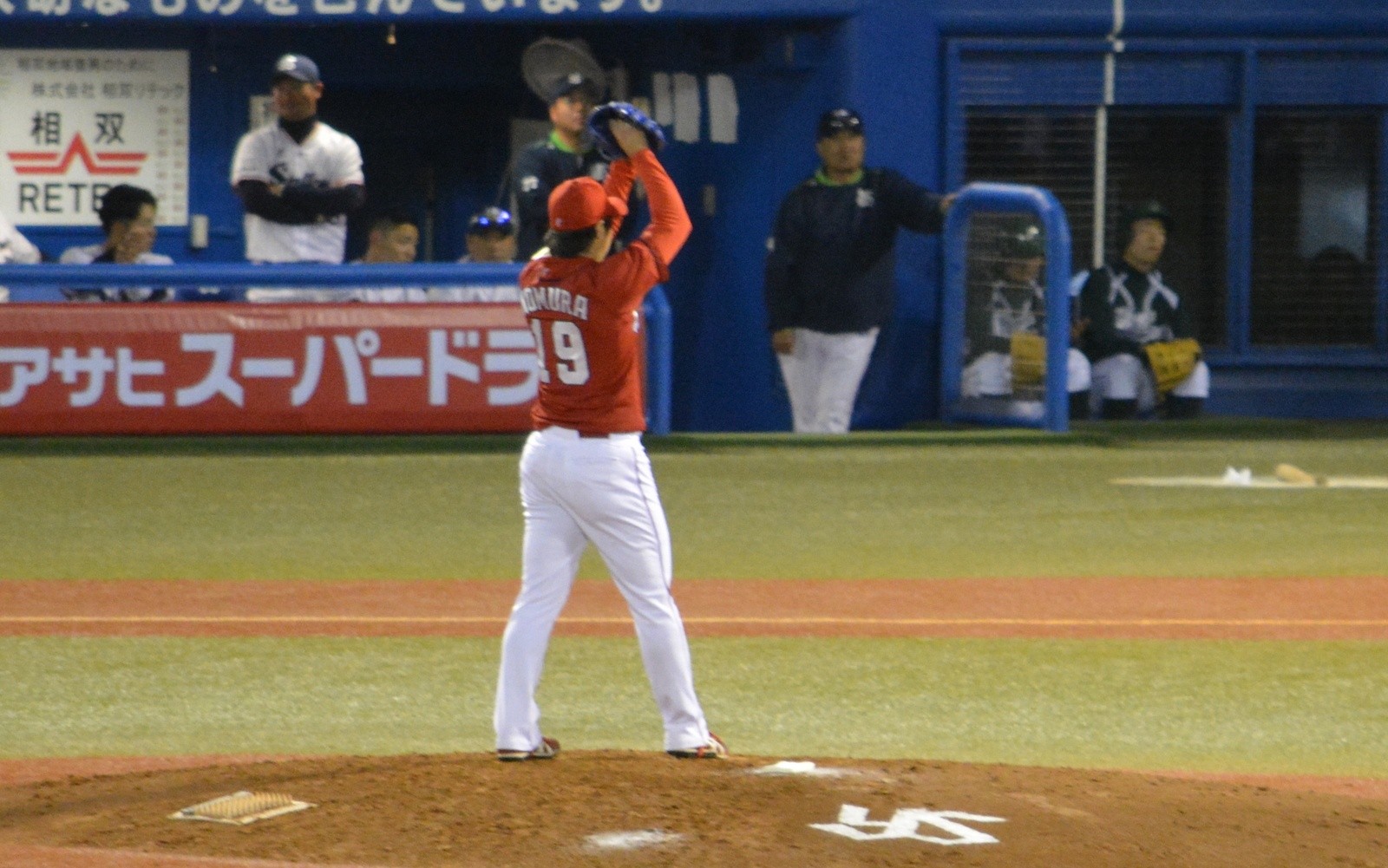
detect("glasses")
[468,217,512,235]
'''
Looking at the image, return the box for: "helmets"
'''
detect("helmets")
[1115,201,1174,252]
[1000,224,1044,257]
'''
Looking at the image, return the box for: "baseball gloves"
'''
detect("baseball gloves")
[1144,336,1203,393]
[585,100,667,161]
[1009,331,1047,386]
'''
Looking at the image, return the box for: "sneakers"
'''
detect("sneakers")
[496,738,559,760]
[669,732,727,759]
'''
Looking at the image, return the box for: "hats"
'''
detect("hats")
[547,178,629,232]
[811,106,865,146]
[272,53,320,82]
[550,75,594,97]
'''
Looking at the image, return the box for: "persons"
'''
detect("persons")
[59,184,176,303]
[331,198,428,304]
[1071,203,1210,420]
[962,219,1090,421]
[230,54,367,303]
[765,109,958,431]
[0,210,40,303]
[514,71,612,261]
[493,120,727,760]
[427,206,519,302]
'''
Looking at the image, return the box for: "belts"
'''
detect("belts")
[533,423,608,438]
